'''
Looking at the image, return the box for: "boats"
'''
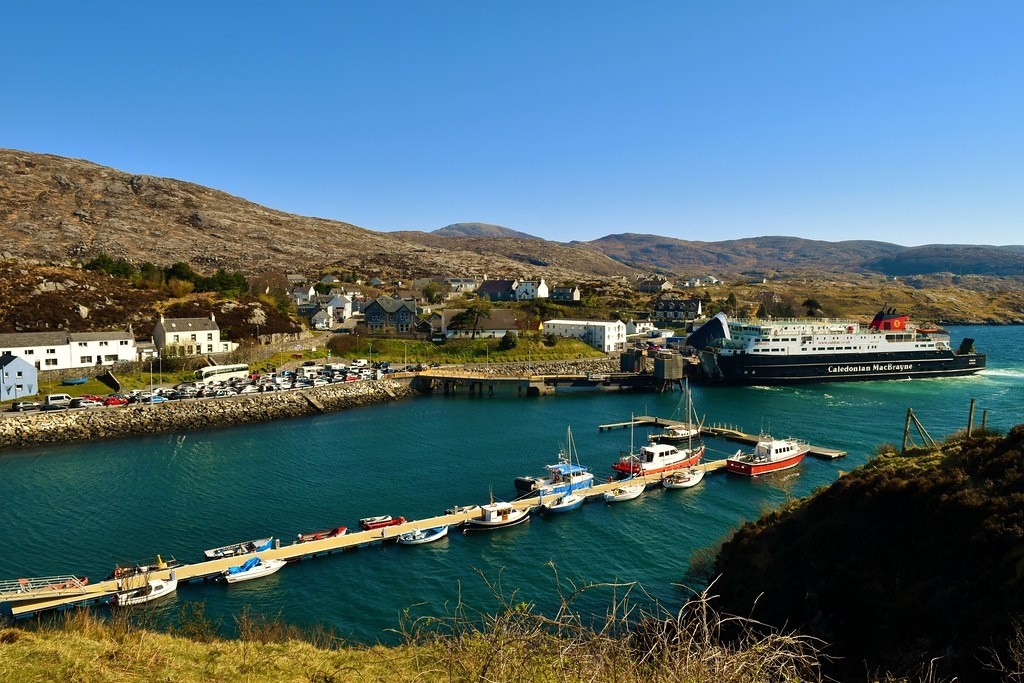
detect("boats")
[298,526,347,543]
[435,503,480,517]
[462,480,532,533]
[516,439,595,498]
[659,389,705,489]
[724,416,812,478]
[106,571,178,606]
[359,515,407,530]
[215,557,288,584]
[203,535,273,559]
[395,525,448,545]
[37,577,88,591]
[107,554,181,581]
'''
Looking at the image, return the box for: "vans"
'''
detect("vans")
[43,393,73,405]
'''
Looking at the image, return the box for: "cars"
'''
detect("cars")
[39,404,68,411]
[71,352,442,410]
[12,400,41,411]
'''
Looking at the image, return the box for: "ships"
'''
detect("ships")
[692,307,987,384]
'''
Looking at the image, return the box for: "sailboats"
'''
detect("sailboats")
[601,412,646,503]
[641,385,706,442]
[609,375,705,472]
[538,424,584,511]
